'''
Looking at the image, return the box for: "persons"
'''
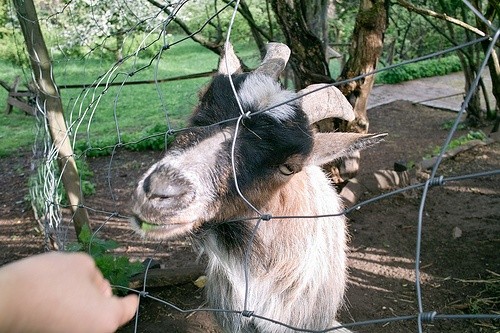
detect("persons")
[1,240,141,333]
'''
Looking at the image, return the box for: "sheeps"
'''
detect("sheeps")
[124,39,389,333]
